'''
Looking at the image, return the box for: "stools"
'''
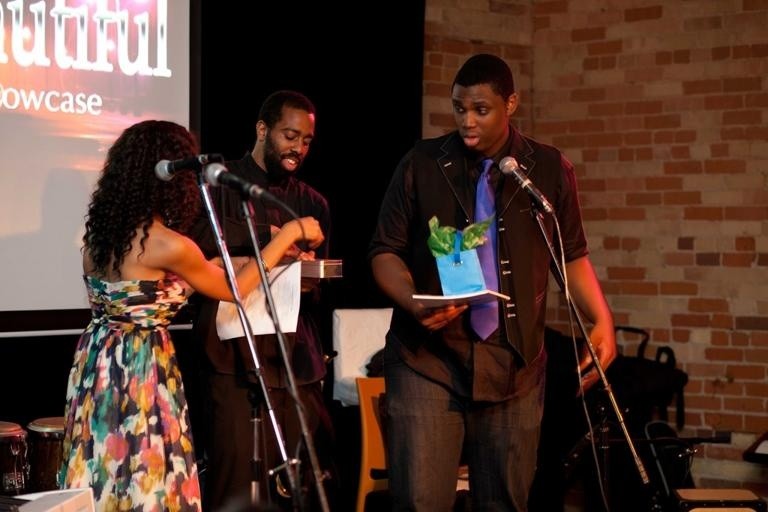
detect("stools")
[678,487,762,512]
[0,421,26,497]
[29,417,65,491]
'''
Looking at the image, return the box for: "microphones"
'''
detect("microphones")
[156,152,224,180]
[499,157,555,216]
[204,163,275,207]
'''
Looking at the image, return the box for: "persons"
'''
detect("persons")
[57,120,324,511]
[190,93,330,512]
[369,54,617,512]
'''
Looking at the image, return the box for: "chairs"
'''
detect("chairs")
[354,375,471,512]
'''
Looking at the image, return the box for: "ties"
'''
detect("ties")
[466,155,502,344]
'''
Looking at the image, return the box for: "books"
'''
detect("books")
[412,292,511,308]
